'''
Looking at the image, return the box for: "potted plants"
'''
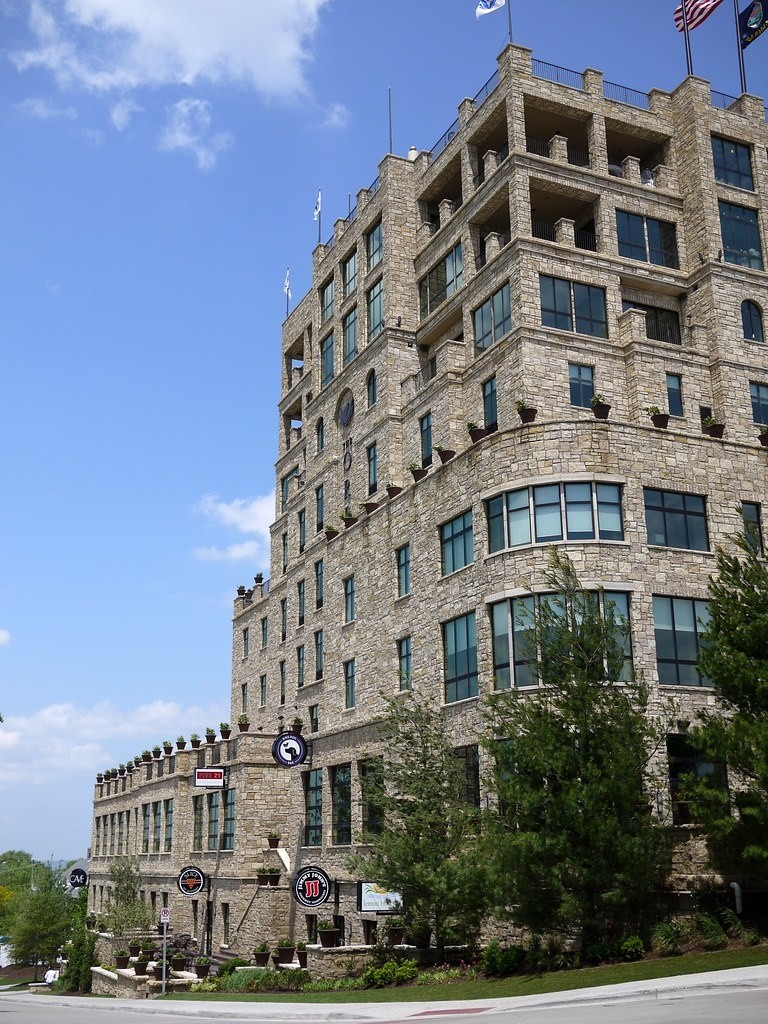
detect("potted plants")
[128,939,141,957]
[339,511,358,529]
[431,445,456,465]
[133,954,150,975]
[193,956,211,979]
[645,406,670,429]
[141,750,151,762]
[159,948,173,966]
[385,481,403,500]
[590,394,611,419]
[126,761,134,773]
[291,717,303,735]
[237,713,250,732]
[295,941,315,968]
[406,463,428,483]
[245,589,253,599]
[114,949,130,969]
[317,919,340,948]
[103,770,111,781]
[257,867,269,885]
[253,941,271,967]
[237,586,246,596]
[96,773,103,783]
[142,942,155,961]
[134,756,143,767]
[190,733,201,748]
[171,953,188,971]
[702,416,725,439]
[515,399,537,424]
[205,728,216,744]
[163,741,173,755]
[152,959,170,981]
[96,919,108,933]
[111,768,118,778]
[219,722,232,739]
[176,736,187,750]
[118,764,126,776]
[413,922,433,949]
[757,428,768,446]
[152,745,162,758]
[267,832,280,848]
[157,917,169,935]
[359,501,380,515]
[267,868,281,886]
[467,422,487,444]
[383,917,405,945]
[254,572,264,584]
[324,525,339,542]
[271,937,296,965]
[85,915,96,930]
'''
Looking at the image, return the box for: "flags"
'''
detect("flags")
[283,270,291,300]
[314,191,321,220]
[673,0,768,50]
[475,0,505,20]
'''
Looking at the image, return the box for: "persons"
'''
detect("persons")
[44,966,56,984]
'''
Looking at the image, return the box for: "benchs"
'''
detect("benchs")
[28,983,52,993]
